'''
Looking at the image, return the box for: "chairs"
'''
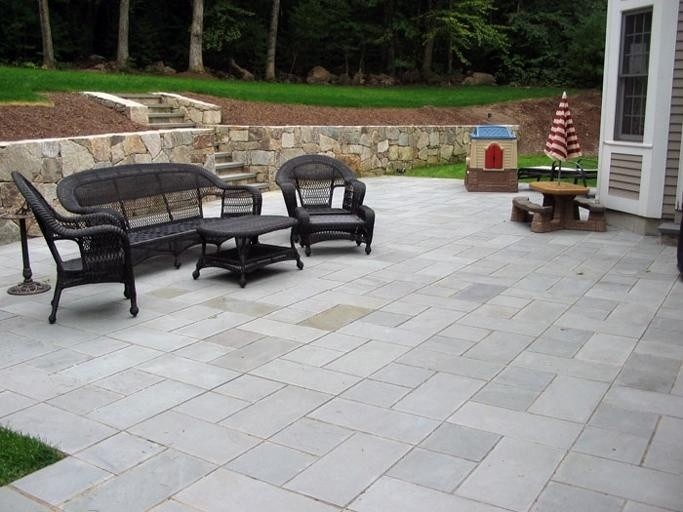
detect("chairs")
[11,170,139,325]
[518,158,598,198]
[275,155,375,257]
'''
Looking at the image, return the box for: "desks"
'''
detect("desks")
[531,181,589,230]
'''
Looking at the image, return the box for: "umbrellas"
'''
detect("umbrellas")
[542,91,582,186]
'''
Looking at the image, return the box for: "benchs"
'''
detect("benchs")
[57,163,262,269]
[574,198,607,232]
[510,197,553,233]
